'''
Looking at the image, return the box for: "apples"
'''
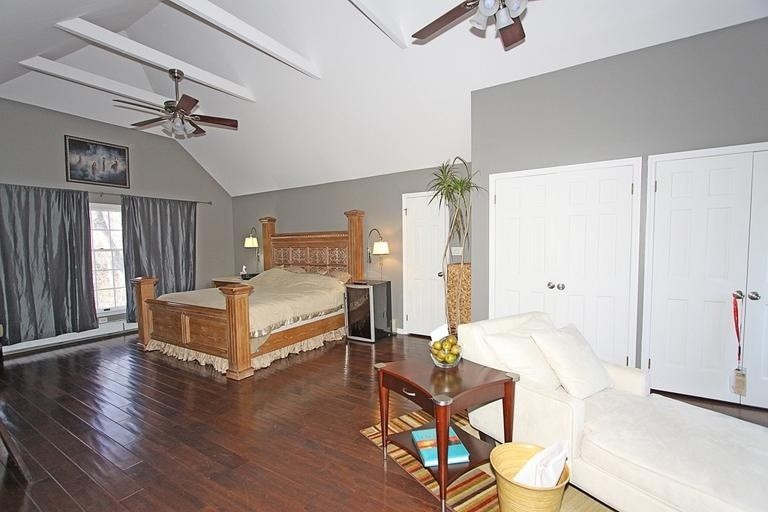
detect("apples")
[430,335,461,364]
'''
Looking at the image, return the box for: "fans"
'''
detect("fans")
[409,1,527,48]
[112,70,238,135]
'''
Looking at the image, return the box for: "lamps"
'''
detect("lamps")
[161,110,196,135]
[367,228,388,270]
[468,1,527,31]
[243,226,260,274]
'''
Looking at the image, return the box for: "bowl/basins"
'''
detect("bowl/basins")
[428,340,465,369]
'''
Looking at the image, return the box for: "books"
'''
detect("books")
[411,425,471,468]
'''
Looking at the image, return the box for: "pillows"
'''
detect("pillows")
[273,260,352,285]
[478,315,649,397]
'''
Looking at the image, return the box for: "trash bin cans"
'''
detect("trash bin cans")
[490,442,569,512]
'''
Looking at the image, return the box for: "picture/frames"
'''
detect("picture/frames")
[63,135,132,189]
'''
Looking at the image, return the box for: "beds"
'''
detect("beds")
[456,311,768,510]
[130,209,366,381]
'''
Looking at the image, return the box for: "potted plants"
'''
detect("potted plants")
[426,156,494,332]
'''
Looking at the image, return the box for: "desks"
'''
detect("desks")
[378,351,522,512]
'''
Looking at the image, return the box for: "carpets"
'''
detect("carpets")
[359,398,618,512]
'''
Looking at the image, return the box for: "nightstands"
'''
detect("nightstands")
[345,279,391,344]
[210,274,243,288]
